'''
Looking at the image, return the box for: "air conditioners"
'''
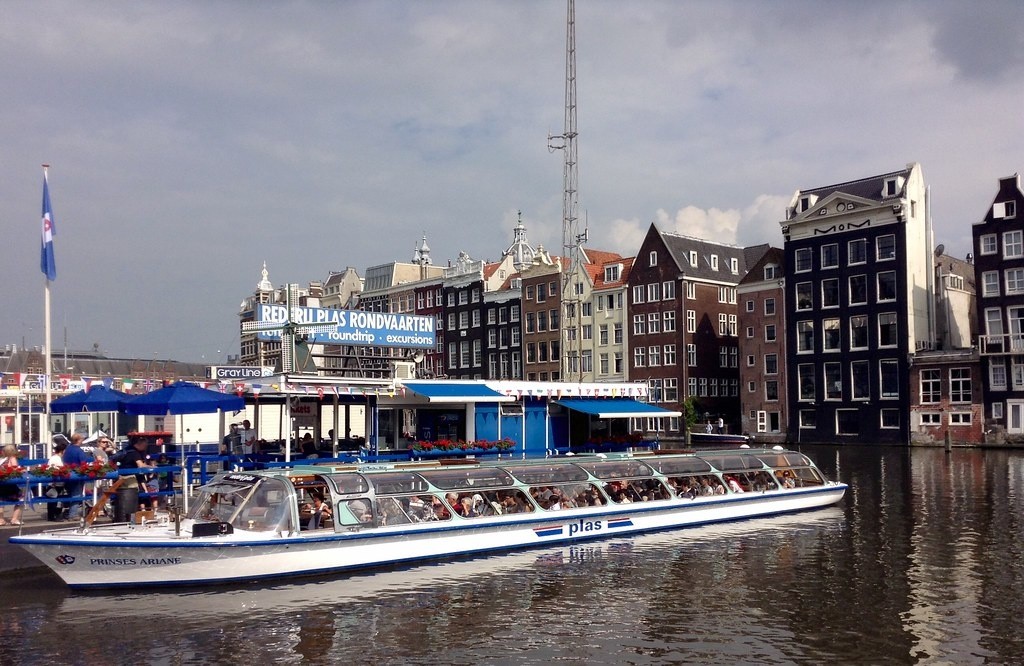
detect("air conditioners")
[390,361,415,379]
[926,341,934,350]
[917,340,927,350]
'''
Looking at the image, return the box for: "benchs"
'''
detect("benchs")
[0,465,183,509]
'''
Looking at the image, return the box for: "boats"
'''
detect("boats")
[8,448,848,592]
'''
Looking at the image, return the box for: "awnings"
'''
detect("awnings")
[555,398,682,419]
[403,383,516,403]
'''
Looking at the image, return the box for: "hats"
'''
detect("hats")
[54,443,67,450]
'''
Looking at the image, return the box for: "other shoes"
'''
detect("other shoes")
[10,519,24,526]
[0,520,10,526]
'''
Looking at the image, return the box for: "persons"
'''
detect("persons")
[221,419,255,465]
[62,433,95,522]
[616,490,631,504]
[546,495,560,512]
[698,476,714,496]
[705,421,713,434]
[714,417,724,435]
[0,445,24,525]
[300,470,796,531]
[300,429,333,459]
[680,482,695,499]
[90,437,179,521]
[461,497,475,517]
[45,445,64,522]
[442,493,462,518]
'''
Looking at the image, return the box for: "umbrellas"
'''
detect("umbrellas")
[49,384,138,441]
[117,381,246,469]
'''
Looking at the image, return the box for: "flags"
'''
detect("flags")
[40,177,58,282]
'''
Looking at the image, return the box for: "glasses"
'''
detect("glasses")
[423,508,428,511]
[101,441,108,443]
